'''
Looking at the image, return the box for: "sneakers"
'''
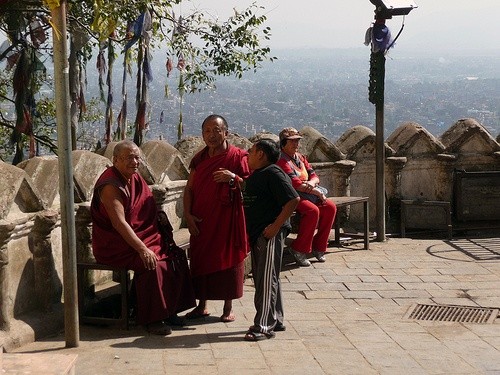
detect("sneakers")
[311,249,326,262]
[289,246,312,267]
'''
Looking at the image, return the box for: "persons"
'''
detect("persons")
[183,114,253,321]
[242,138,301,341]
[90,141,196,336]
[276,127,336,266]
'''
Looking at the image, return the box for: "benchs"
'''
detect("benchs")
[76,196,371,331]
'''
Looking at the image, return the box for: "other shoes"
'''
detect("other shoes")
[146,320,170,335]
[166,314,186,326]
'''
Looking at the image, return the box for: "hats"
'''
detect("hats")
[279,126,303,142]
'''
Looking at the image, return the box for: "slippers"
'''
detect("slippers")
[185,308,211,319]
[222,311,235,321]
[244,331,269,340]
[250,323,286,332]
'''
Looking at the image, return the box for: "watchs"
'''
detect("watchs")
[229,175,236,186]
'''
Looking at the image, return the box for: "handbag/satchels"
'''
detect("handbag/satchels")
[298,184,328,204]
